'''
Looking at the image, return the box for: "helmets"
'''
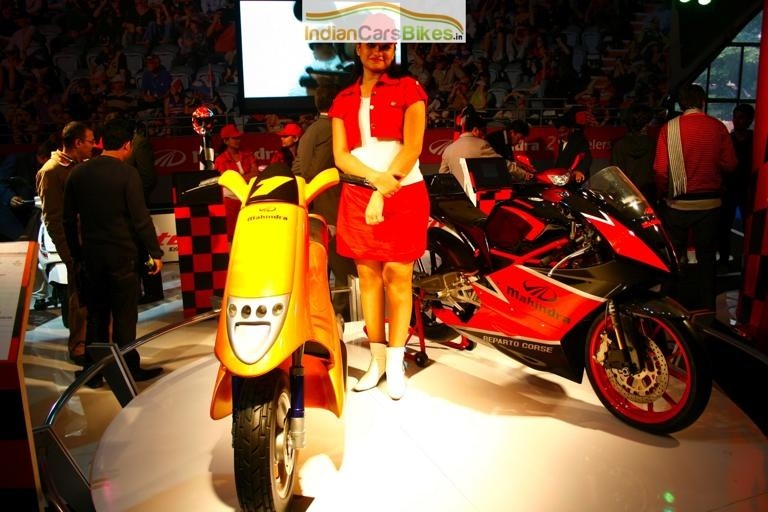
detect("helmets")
[191,107,214,135]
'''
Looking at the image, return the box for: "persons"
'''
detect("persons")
[0,1,767,387]
[328,15,434,403]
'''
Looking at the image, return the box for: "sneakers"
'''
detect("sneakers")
[74,370,105,389]
[131,365,164,381]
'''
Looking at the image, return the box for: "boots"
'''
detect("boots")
[386,345,406,400]
[353,343,387,393]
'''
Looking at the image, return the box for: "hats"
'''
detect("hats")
[275,124,303,136]
[220,124,244,139]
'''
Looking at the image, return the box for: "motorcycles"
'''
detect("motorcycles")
[176,165,376,511]
[404,159,710,434]
[513,150,591,204]
[19,196,74,331]
